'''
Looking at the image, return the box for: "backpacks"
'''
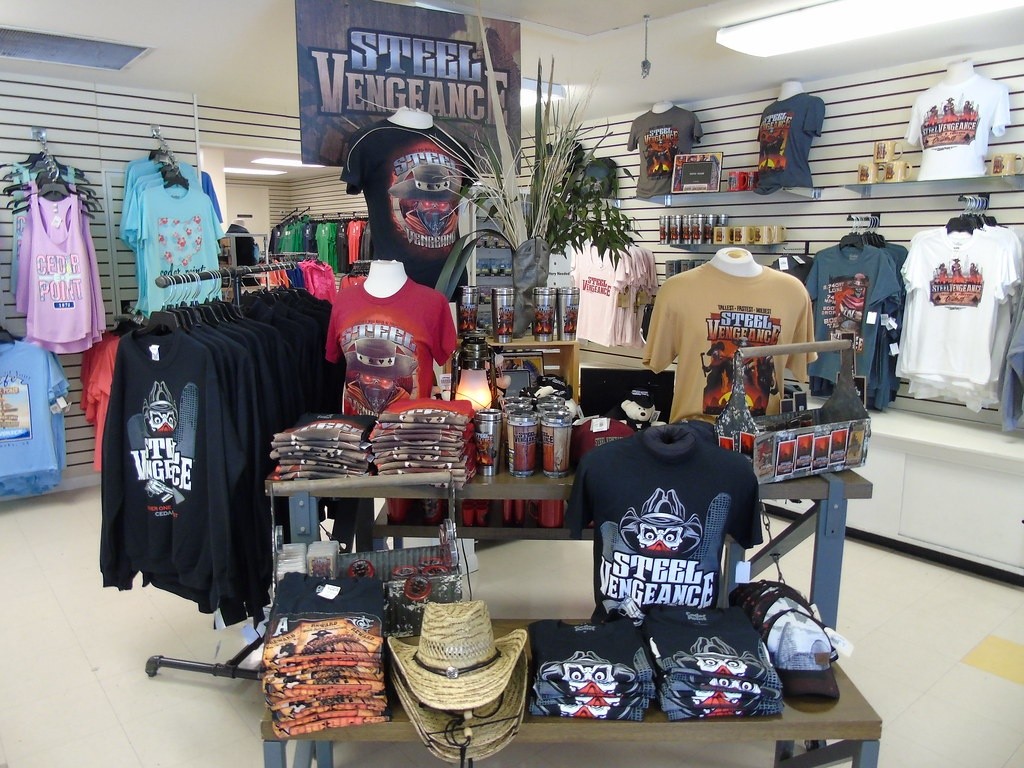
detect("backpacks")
[577,157,616,200]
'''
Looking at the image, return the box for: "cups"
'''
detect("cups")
[456,285,478,333]
[559,286,581,341]
[657,214,728,244]
[727,170,760,193]
[491,286,516,343]
[473,408,503,477]
[530,286,557,342]
[990,152,1024,177]
[502,393,570,477]
[857,140,913,185]
[714,225,787,246]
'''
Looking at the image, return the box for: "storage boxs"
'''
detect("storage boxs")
[666,259,705,280]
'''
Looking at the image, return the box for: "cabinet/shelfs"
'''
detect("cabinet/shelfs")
[629,184,844,251]
[457,188,531,311]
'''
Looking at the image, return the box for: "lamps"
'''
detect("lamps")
[715,0,1024,56]
[450,330,500,411]
[520,77,566,110]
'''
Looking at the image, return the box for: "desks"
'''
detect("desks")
[261,619,882,768]
[266,437,872,630]
[443,328,579,406]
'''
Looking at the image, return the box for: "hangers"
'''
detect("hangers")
[0,137,102,219]
[109,305,148,337]
[347,260,374,278]
[260,251,325,268]
[839,216,886,250]
[148,133,189,190]
[0,325,23,345]
[131,267,243,337]
[232,262,319,313]
[946,196,997,234]
[276,209,369,232]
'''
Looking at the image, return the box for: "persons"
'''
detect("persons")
[905,58,1011,180]
[627,101,704,198]
[562,426,764,621]
[755,81,826,194]
[643,247,818,424]
[324,261,457,416]
[339,106,476,304]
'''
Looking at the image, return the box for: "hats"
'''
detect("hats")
[386,600,529,764]
[729,578,842,703]
